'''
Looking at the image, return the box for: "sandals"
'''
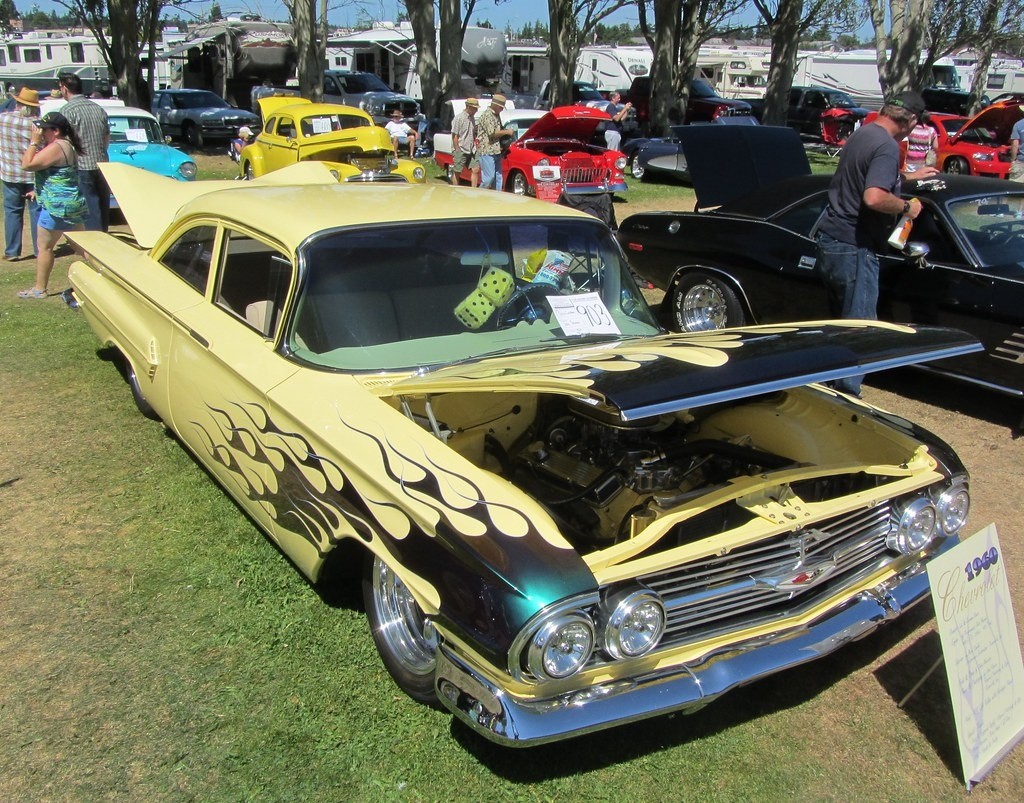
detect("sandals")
[17,287,49,299]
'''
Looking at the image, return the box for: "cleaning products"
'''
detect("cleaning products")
[886,197,924,251]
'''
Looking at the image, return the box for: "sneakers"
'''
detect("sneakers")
[2,254,19,262]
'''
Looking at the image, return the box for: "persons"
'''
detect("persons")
[0,87,44,261]
[233,127,256,162]
[18,112,91,298]
[475,94,515,191]
[1008,118,1024,218]
[814,90,940,400]
[57,72,111,233]
[905,110,938,173]
[385,110,419,160]
[450,98,481,187]
[604,91,633,152]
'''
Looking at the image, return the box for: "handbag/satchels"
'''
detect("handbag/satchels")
[500,127,512,150]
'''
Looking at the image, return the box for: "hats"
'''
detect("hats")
[8,87,44,107]
[887,91,927,130]
[239,126,254,135]
[465,97,481,108]
[390,110,404,118]
[33,112,69,128]
[491,94,507,111]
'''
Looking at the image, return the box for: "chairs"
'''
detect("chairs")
[226,250,501,357]
[911,209,957,264]
[382,122,411,156]
[291,119,317,139]
[227,152,248,182]
[182,94,200,109]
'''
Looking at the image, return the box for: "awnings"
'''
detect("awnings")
[156,36,215,62]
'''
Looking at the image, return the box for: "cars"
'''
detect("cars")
[0,91,55,114]
[855,101,1024,180]
[35,98,197,212]
[922,89,1024,119]
[238,96,427,190]
[59,159,976,748]
[614,124,1023,400]
[431,97,632,204]
[154,88,262,147]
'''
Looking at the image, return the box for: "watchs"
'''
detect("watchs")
[30,142,37,146]
[903,201,910,212]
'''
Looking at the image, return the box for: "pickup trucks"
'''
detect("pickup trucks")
[598,76,753,124]
[251,69,423,139]
[736,85,867,148]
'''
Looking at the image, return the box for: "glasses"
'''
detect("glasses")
[392,115,398,117]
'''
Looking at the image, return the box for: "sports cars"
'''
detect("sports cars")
[623,116,761,185]
